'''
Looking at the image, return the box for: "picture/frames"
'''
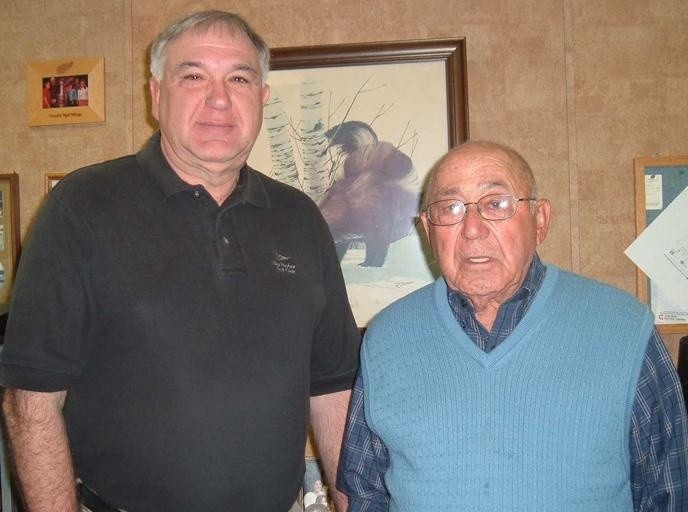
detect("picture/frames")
[40,173,66,193]
[20,55,108,127]
[0,170,23,321]
[245,34,470,338]
[297,454,337,511]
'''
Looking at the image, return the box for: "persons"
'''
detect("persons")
[43,77,88,108]
[1,10,364,511]
[336,142,688,510]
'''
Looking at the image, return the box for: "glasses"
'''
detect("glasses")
[423,192,538,228]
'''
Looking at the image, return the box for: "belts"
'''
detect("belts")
[73,473,128,512]
